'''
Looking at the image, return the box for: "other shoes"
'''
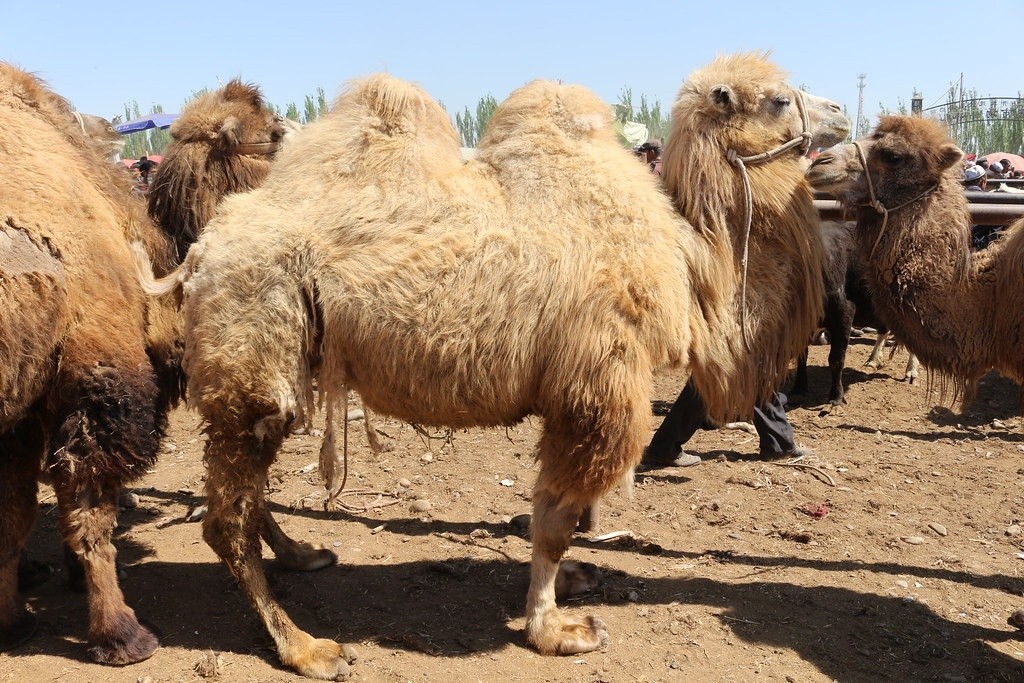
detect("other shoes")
[646,451,702,467]
[760,444,812,460]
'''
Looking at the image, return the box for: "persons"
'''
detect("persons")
[638,140,663,176]
[644,374,812,467]
[116,156,159,180]
[961,153,1024,251]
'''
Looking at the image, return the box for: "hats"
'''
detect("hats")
[965,165,985,181]
[967,154,976,161]
[967,161,976,169]
[639,140,662,153]
[976,156,988,164]
[992,162,1004,173]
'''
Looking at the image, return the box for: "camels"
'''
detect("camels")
[0,59,290,666]
[788,220,920,416]
[130,49,851,682]
[803,113,1024,420]
